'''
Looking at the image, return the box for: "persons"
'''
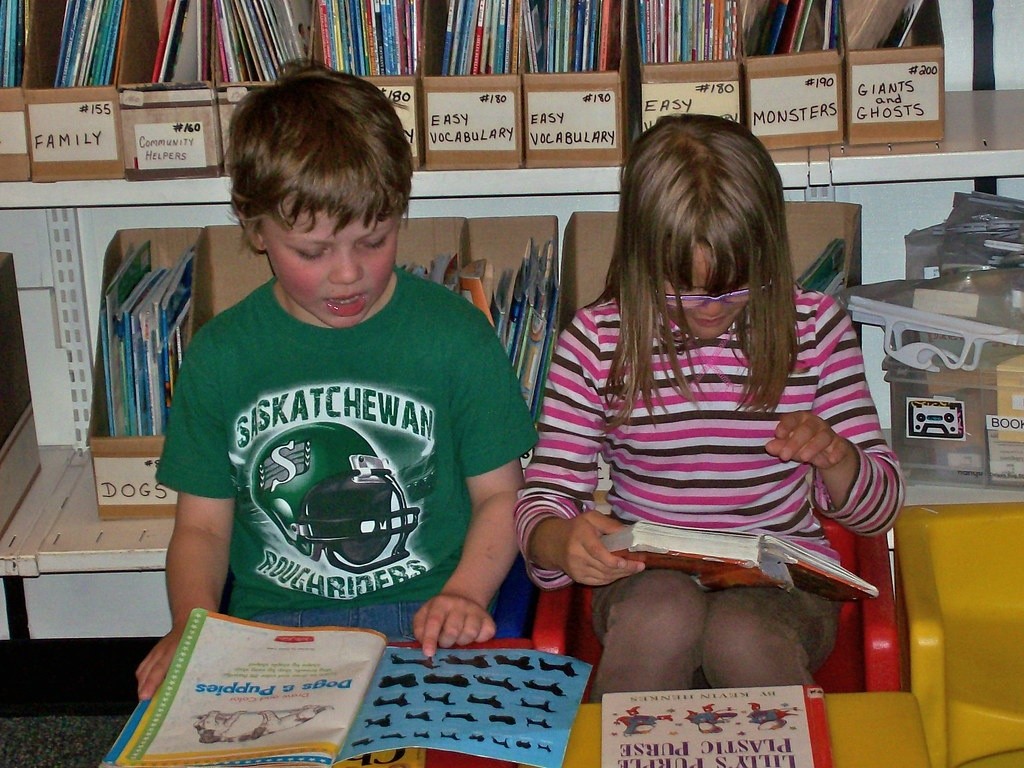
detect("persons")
[508,113,906,692]
[134,65,539,698]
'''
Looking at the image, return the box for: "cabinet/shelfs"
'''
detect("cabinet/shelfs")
[0,91,1022,641]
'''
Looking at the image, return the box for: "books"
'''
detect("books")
[597,521,879,606]
[1,0,849,88]
[95,239,197,435]
[850,0,925,51]
[795,234,852,297]
[102,603,594,765]
[408,240,561,473]
[597,685,836,768]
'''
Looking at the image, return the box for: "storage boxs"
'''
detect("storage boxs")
[0,1,944,183]
[845,269,1024,488]
[88,204,863,520]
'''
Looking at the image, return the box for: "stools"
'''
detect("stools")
[535,509,902,695]
[893,505,1023,768]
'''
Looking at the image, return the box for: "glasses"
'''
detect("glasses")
[655,288,765,308]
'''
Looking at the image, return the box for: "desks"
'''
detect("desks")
[518,693,931,767]
